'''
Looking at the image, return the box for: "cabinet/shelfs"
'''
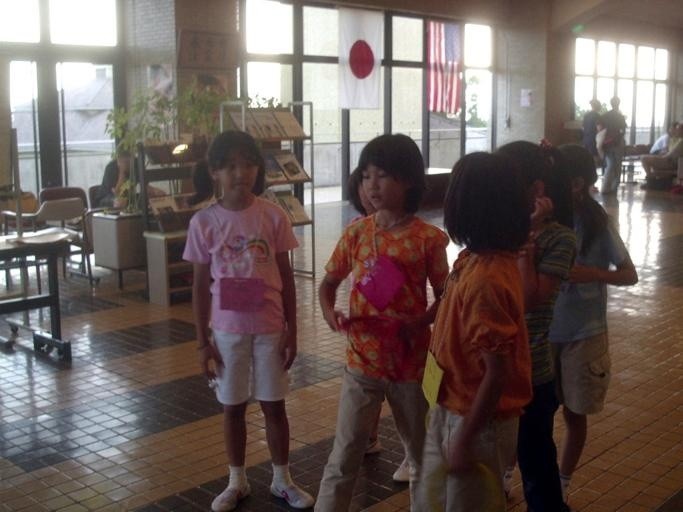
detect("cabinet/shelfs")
[143,230,190,308]
[220,101,316,278]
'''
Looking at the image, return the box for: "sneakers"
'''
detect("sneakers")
[210,483,252,512]
[268,480,315,509]
[392,457,411,482]
[365,438,382,454]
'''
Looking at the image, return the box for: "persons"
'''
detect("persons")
[583,97,683,194]
[183,130,314,512]
[346,166,410,482]
[493,141,578,512]
[503,144,638,499]
[313,133,449,512]
[413,151,532,512]
[97,151,166,208]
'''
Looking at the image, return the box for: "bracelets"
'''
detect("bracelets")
[197,340,210,350]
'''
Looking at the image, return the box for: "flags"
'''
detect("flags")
[425,18,463,115]
[338,5,384,111]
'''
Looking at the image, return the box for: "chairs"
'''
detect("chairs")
[1,185,166,364]
[596,144,683,191]
